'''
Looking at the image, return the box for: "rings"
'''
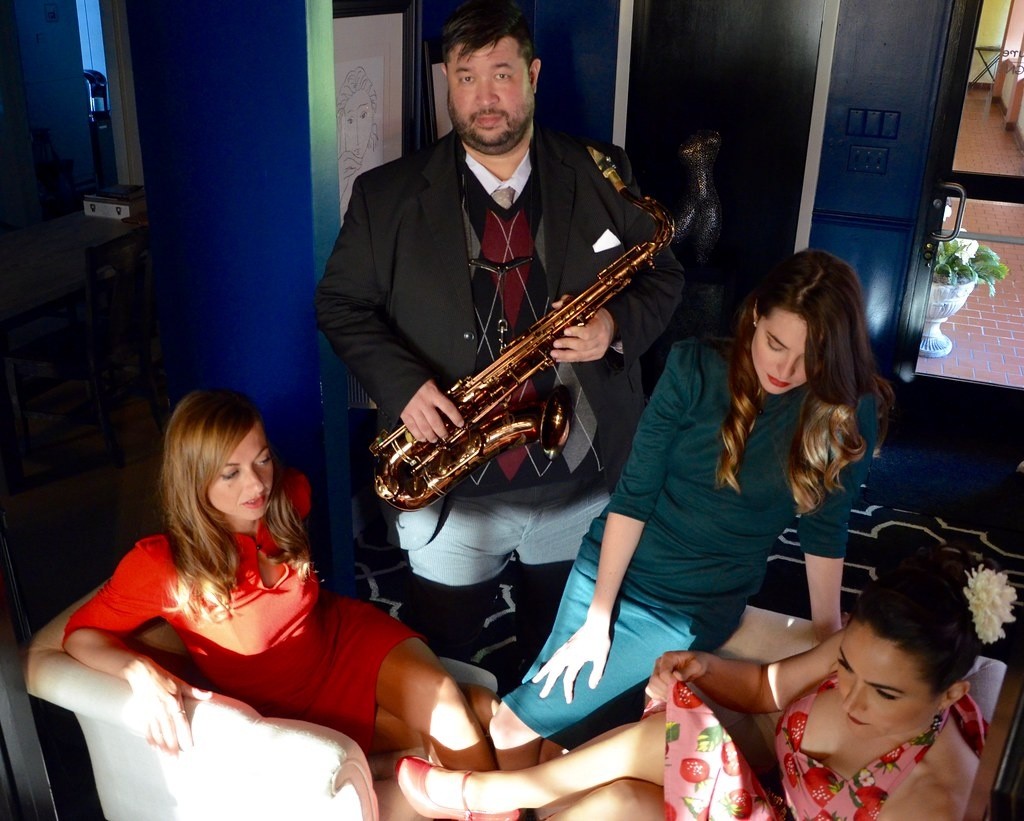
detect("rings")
[173,710,186,719]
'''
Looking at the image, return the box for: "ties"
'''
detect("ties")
[491,186,515,209]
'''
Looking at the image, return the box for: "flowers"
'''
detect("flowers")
[963,563,1017,644]
[934,205,1009,298]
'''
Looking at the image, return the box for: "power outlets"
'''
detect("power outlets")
[847,146,889,175]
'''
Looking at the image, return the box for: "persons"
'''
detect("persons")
[315,0,684,692]
[61,387,501,773]
[488,246,893,770]
[397,566,982,821]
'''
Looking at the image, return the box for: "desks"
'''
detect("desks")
[0,211,154,496]
[968,46,1001,91]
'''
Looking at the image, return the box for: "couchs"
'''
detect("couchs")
[18,579,498,821]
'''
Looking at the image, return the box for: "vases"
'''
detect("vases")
[919,281,976,358]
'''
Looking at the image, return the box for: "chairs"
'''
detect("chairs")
[14,227,162,470]
[645,602,1024,821]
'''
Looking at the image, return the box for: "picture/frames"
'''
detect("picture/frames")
[424,38,453,144]
[332,0,423,227]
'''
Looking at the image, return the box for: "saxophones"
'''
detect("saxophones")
[368,143,675,511]
